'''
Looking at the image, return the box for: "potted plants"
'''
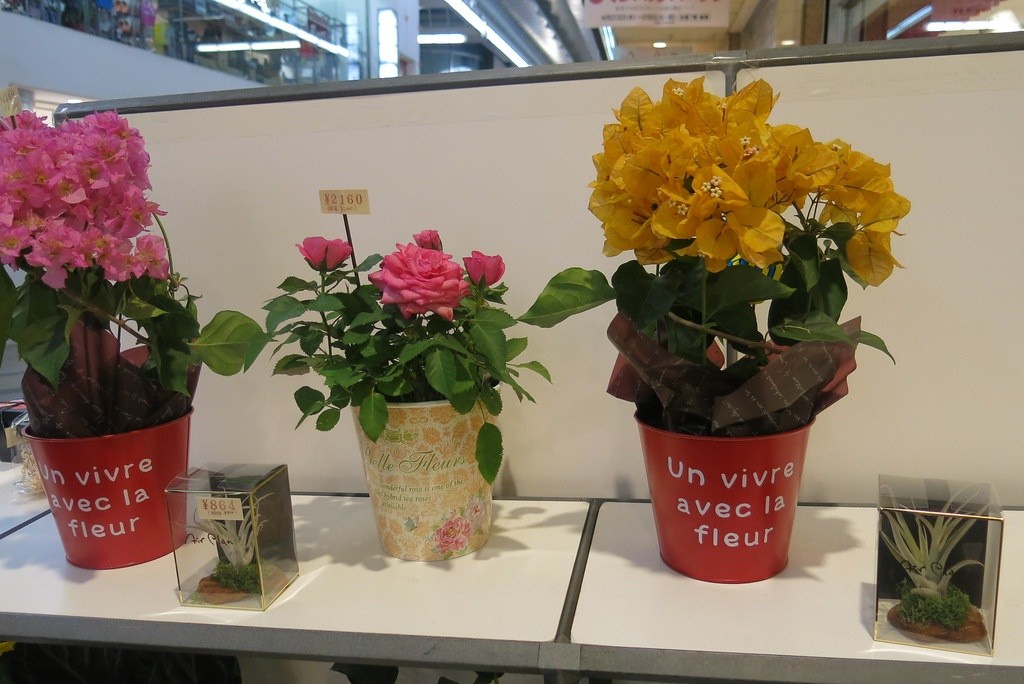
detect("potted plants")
[164,462,300,613]
[875,473,1002,653]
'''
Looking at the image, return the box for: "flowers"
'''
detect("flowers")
[270,230,557,443]
[514,64,907,439]
[1,110,274,438]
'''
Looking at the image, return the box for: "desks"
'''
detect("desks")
[0,459,1024,684]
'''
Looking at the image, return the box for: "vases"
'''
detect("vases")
[635,408,812,585]
[345,398,493,559]
[27,406,193,570]
